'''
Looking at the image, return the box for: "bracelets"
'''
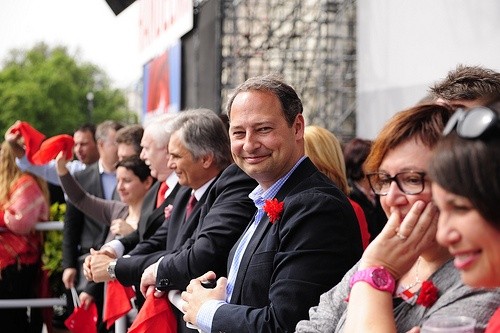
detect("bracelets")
[107,259,116,280]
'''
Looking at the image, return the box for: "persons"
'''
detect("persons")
[0,63,500,333]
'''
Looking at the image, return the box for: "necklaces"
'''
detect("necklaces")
[414,257,451,286]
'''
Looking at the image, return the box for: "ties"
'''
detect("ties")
[186,195,197,221]
[156,181,169,208]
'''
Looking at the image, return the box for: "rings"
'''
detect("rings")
[397,232,407,240]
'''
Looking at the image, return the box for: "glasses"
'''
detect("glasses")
[367,171,427,196]
[441,107,500,138]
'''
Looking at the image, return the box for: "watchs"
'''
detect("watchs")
[349,264,399,296]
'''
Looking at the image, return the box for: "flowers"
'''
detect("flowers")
[164,203,173,220]
[416,281,439,307]
[262,199,283,223]
